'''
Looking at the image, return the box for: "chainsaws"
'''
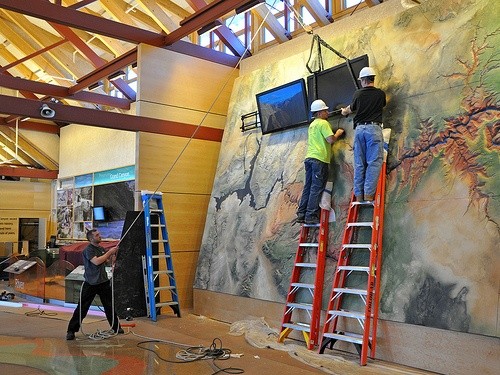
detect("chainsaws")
[0,291,15,301]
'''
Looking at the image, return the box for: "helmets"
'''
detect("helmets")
[358,67,376,81]
[311,100,329,112]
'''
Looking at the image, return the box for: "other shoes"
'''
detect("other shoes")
[116,328,124,334]
[297,218,305,223]
[364,195,374,201]
[67,331,74,340]
[306,219,319,224]
[356,195,363,201]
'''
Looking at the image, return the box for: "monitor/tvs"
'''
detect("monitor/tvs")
[308,54,368,119]
[93,206,107,221]
[255,78,309,135]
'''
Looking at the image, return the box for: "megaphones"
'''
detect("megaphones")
[41,104,56,118]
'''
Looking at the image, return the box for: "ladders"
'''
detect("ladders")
[319,129,391,366]
[142,255,152,318]
[277,182,332,351]
[141,190,180,321]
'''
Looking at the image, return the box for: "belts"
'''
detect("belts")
[357,121,381,128]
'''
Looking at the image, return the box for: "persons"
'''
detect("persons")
[65,230,125,340]
[295,100,345,223]
[342,67,386,203]
